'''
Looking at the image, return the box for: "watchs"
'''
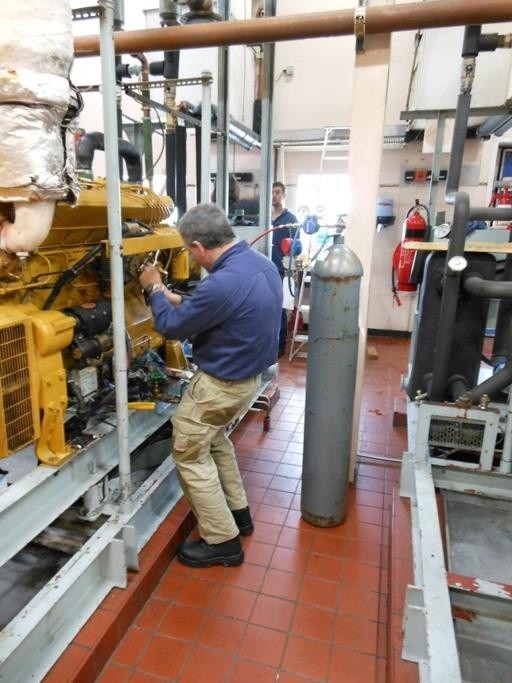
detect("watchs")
[146,283,162,297]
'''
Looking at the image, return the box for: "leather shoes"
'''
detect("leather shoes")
[177,538,244,567]
[277,341,285,357]
[231,506,253,536]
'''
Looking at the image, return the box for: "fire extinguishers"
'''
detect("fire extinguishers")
[390,203,431,308]
[486,187,512,232]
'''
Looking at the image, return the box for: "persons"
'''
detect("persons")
[271,182,300,359]
[139,204,283,568]
